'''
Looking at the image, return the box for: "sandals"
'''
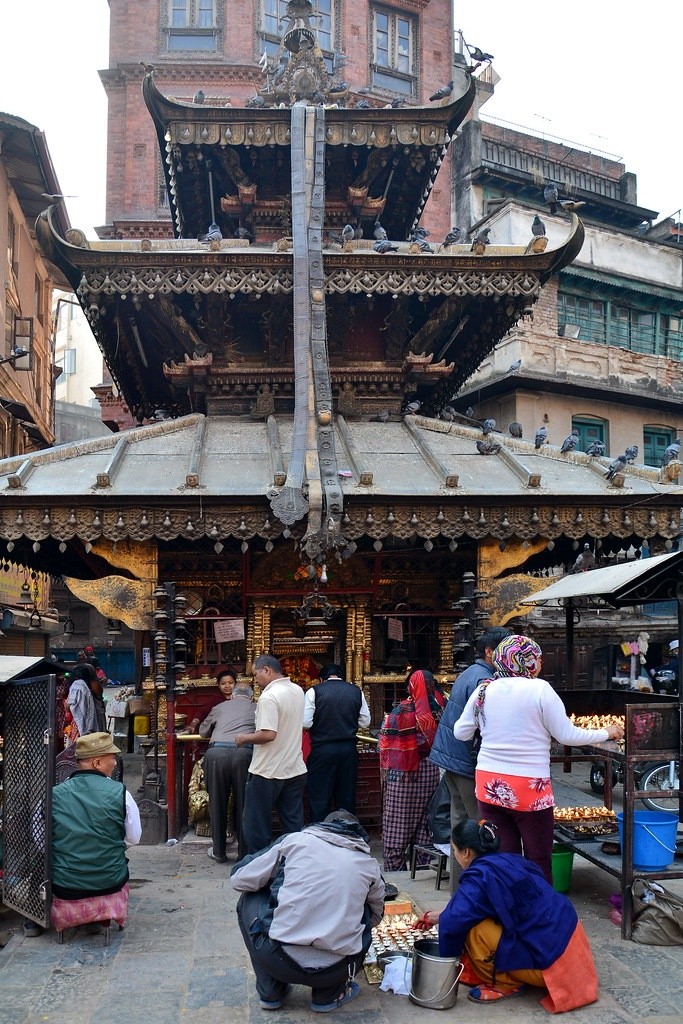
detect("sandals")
[467,983,527,1004]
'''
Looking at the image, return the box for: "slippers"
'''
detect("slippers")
[310,981,361,1013]
[259,983,291,1010]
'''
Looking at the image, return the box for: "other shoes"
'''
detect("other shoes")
[206,846,227,863]
[23,920,42,937]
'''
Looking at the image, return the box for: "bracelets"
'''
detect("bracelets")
[423,911,434,926]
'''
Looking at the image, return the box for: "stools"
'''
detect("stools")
[47,884,130,945]
[412,845,450,890]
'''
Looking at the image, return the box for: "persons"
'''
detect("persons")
[414,819,598,1014]
[453,636,625,887]
[22,732,142,937]
[377,670,448,871]
[55,647,109,754]
[230,810,386,1013]
[429,627,515,897]
[667,640,682,677]
[197,655,371,864]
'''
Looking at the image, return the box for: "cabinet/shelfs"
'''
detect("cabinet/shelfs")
[549,688,683,939]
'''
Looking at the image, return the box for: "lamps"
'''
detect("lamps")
[15,583,34,610]
[562,323,581,339]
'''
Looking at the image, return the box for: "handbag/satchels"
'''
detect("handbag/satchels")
[630,879,682,945]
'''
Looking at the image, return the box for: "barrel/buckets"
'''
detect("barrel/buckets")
[404,938,466,1010]
[616,809,680,872]
[134,715,150,735]
[550,843,574,893]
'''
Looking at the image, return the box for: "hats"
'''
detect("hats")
[74,732,121,759]
[86,646,93,652]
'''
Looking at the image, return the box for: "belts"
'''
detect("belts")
[209,742,253,748]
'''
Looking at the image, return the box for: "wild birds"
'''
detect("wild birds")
[429,81,454,102]
[324,221,492,255]
[465,44,494,63]
[192,90,205,104]
[369,399,683,481]
[245,81,410,109]
[204,222,222,242]
[13,345,23,356]
